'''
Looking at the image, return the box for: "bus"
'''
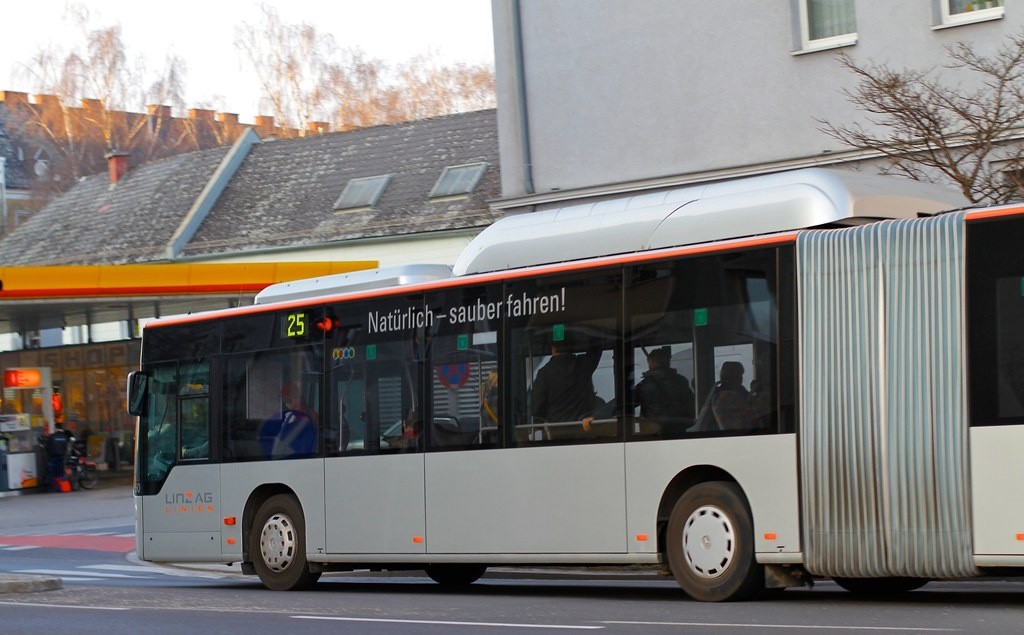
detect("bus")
[125,156,1024,605]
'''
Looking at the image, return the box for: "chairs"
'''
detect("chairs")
[690,381,773,435]
[259,408,315,456]
[418,413,480,450]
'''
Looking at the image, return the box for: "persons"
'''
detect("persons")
[482,370,501,444]
[42,423,67,492]
[388,410,419,449]
[582,346,697,439]
[233,352,281,422]
[716,362,785,435]
[529,329,609,445]
[182,413,249,459]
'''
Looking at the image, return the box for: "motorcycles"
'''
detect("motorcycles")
[64,425,101,490]
